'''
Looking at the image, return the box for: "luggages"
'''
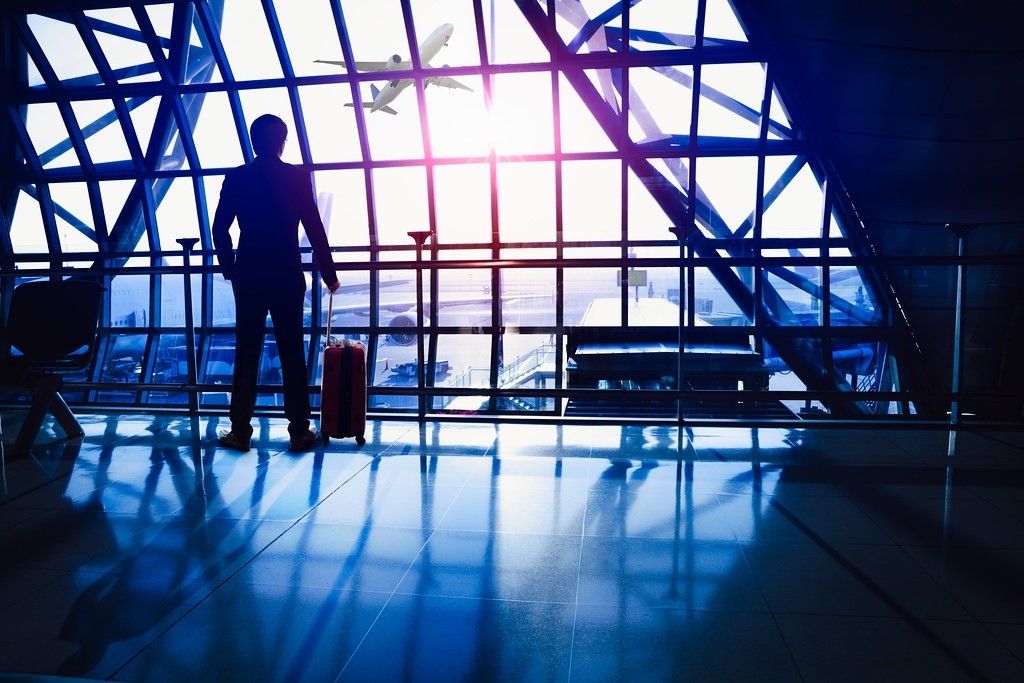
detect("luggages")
[318,287,369,446]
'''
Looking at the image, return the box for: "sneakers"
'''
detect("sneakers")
[220,429,251,452]
[290,428,320,451]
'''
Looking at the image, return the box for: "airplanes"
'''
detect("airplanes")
[312,21,475,116]
[11,183,556,395]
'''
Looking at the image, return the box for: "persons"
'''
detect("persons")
[549,334,554,341]
[414,359,418,375]
[212,114,340,451]
